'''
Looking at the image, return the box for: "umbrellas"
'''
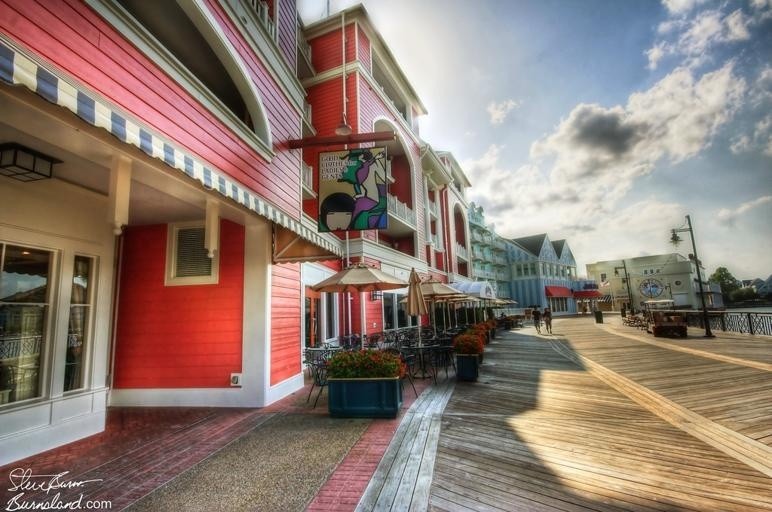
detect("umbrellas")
[307,263,409,349]
[396,266,518,345]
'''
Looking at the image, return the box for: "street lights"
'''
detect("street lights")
[615,260,634,314]
[670,214,714,338]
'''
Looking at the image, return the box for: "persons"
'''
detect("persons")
[531,305,542,335]
[541,307,553,334]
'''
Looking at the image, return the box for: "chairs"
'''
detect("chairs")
[303,324,472,409]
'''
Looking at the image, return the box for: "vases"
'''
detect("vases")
[327,376,403,418]
[456,354,479,381]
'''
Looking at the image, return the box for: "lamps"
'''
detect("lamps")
[0,142,64,182]
[370,291,381,301]
[335,125,353,136]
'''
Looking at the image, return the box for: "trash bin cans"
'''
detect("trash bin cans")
[621,309,625,317]
[594,311,603,323]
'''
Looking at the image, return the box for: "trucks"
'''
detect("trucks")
[639,299,688,339]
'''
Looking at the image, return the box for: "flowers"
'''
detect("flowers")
[452,319,496,353]
